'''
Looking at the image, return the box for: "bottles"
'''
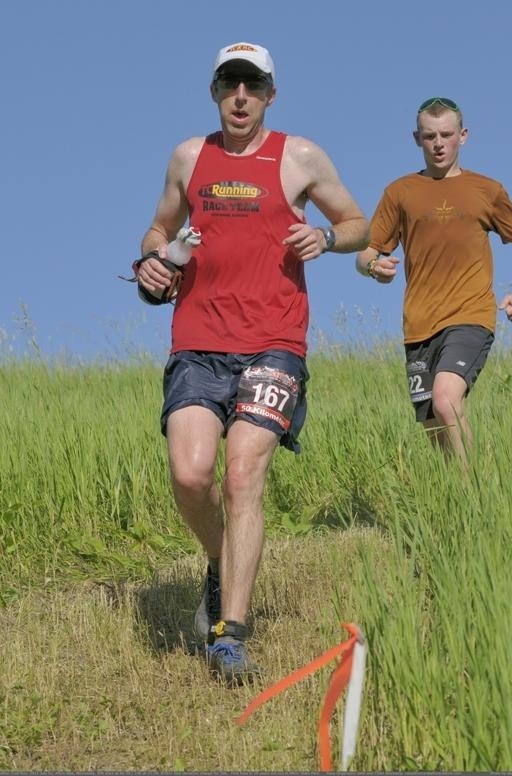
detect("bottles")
[136,223,202,306]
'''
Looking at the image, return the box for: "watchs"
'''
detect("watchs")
[315,225,335,253]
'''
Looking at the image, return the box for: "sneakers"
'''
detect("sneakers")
[212,635,253,687]
[192,565,228,639]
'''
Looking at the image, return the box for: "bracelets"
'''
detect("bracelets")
[366,257,380,280]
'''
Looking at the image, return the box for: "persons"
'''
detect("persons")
[133,40,371,688]
[356,95,512,478]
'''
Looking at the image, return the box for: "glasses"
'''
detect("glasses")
[215,74,269,91]
[212,42,275,88]
[418,97,458,114]
